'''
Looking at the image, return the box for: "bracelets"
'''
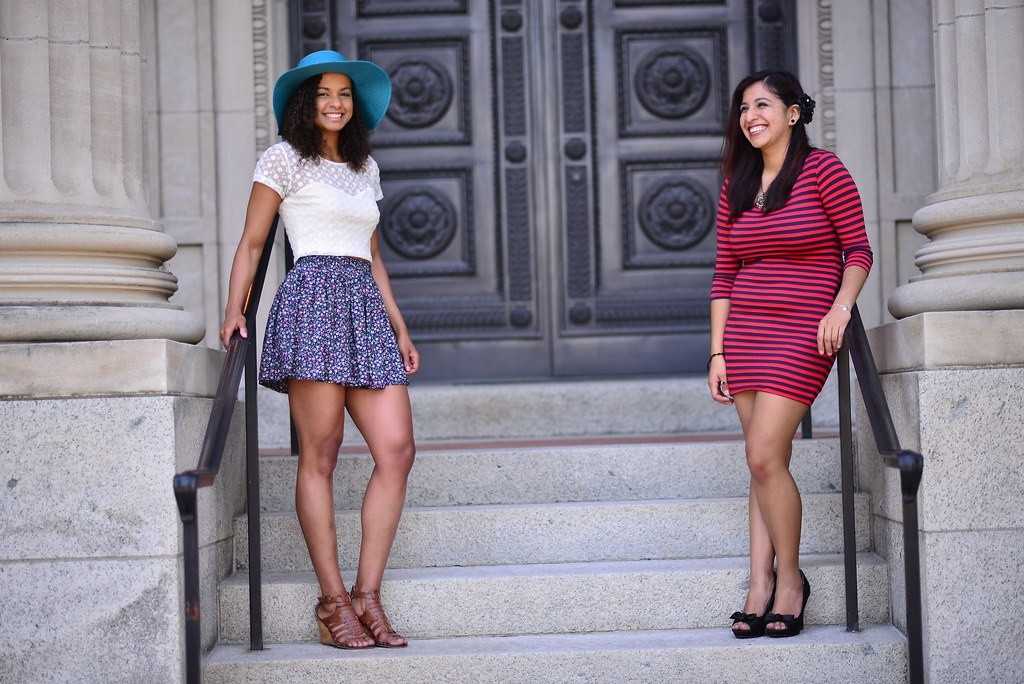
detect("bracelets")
[832,304,852,313]
[707,352,725,368]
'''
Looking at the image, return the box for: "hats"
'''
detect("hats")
[270,49,392,137]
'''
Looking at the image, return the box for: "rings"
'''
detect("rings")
[721,381,727,384]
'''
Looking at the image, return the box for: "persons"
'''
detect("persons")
[706,72,874,639]
[218,51,420,650]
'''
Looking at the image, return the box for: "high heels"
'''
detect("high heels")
[314,594,376,650]
[346,588,410,650]
[731,573,780,640]
[765,567,813,636]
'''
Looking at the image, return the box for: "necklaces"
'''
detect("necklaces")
[755,184,770,209]
